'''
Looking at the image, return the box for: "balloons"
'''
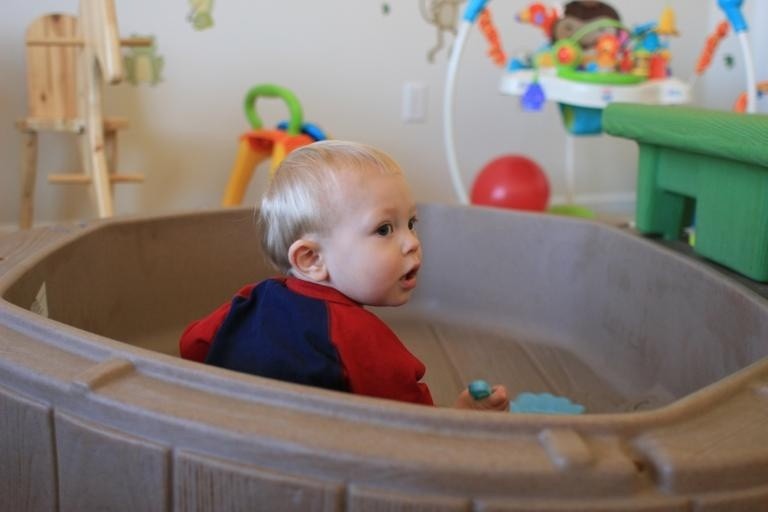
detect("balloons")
[472,156,550,212]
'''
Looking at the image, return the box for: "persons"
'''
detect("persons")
[180,141,510,413]
